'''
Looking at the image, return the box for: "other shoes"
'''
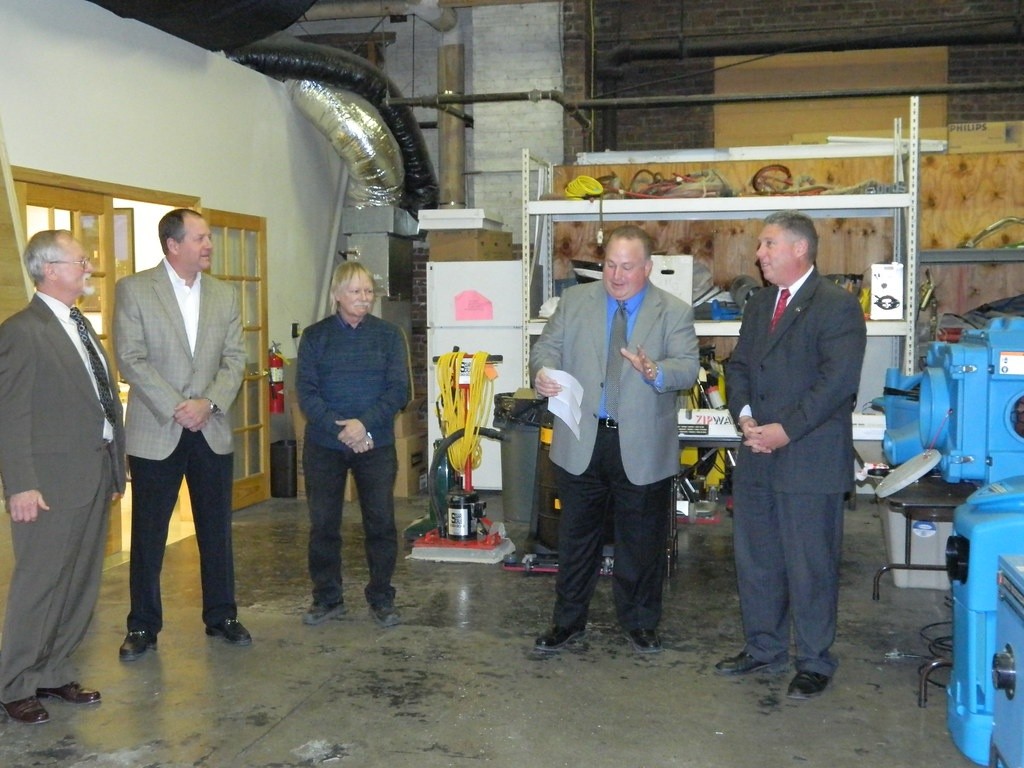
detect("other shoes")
[205,617,252,645]
[370,599,402,626]
[118,628,159,660]
[303,597,347,624]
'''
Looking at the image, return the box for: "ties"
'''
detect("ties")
[70,307,116,427]
[768,288,790,333]
[604,300,628,425]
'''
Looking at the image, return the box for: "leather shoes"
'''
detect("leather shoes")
[786,670,829,698]
[713,651,771,676]
[36,681,103,705]
[622,627,664,653]
[0,695,50,724]
[535,623,586,651]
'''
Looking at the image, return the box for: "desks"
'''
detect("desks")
[870,470,978,600]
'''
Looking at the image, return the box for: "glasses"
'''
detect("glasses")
[48,259,89,270]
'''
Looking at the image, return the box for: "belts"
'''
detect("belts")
[599,418,619,429]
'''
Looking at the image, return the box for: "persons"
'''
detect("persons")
[112,208,252,660]
[0,229,127,724]
[714,209,867,696]
[296,262,413,622]
[534,224,702,650]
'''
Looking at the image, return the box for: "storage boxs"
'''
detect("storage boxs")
[521,96,920,449]
[648,254,694,306]
[883,497,953,591]
[864,263,903,321]
[675,409,741,439]
[290,400,428,503]
[418,211,513,262]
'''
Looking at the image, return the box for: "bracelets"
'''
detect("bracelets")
[654,363,659,377]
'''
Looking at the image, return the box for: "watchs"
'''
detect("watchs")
[206,398,219,415]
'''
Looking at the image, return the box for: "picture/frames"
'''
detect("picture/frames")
[70,208,135,312]
